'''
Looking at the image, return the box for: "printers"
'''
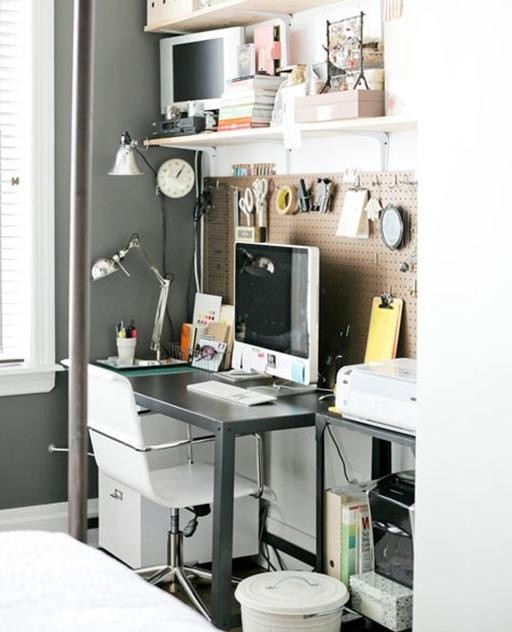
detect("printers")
[335,358,416,436]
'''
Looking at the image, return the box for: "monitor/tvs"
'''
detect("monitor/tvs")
[231,241,322,398]
[160,27,246,115]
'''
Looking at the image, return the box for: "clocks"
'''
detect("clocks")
[156,158,195,198]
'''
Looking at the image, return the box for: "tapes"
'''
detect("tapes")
[276,184,298,214]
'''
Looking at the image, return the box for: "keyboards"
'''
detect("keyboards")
[186,380,277,406]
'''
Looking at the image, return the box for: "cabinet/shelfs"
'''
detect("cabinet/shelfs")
[314,407,415,632]
[143,0,414,173]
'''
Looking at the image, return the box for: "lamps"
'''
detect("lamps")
[91,232,175,366]
[108,130,176,344]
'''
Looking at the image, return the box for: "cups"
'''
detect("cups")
[187,102,206,118]
[164,105,182,120]
[116,336,137,366]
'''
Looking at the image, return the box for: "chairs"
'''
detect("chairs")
[84,363,278,624]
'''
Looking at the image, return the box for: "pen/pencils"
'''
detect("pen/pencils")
[116,319,137,337]
[334,325,351,383]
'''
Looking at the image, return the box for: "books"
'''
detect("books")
[179,292,234,373]
[339,499,375,598]
[216,74,386,133]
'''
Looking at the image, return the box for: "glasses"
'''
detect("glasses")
[194,344,227,362]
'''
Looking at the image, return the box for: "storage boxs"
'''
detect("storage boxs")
[293,89,384,125]
[348,571,414,632]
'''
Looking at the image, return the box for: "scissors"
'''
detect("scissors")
[238,179,269,226]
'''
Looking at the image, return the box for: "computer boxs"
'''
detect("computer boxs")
[366,473,415,593]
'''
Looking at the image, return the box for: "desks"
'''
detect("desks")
[88,361,391,632]
